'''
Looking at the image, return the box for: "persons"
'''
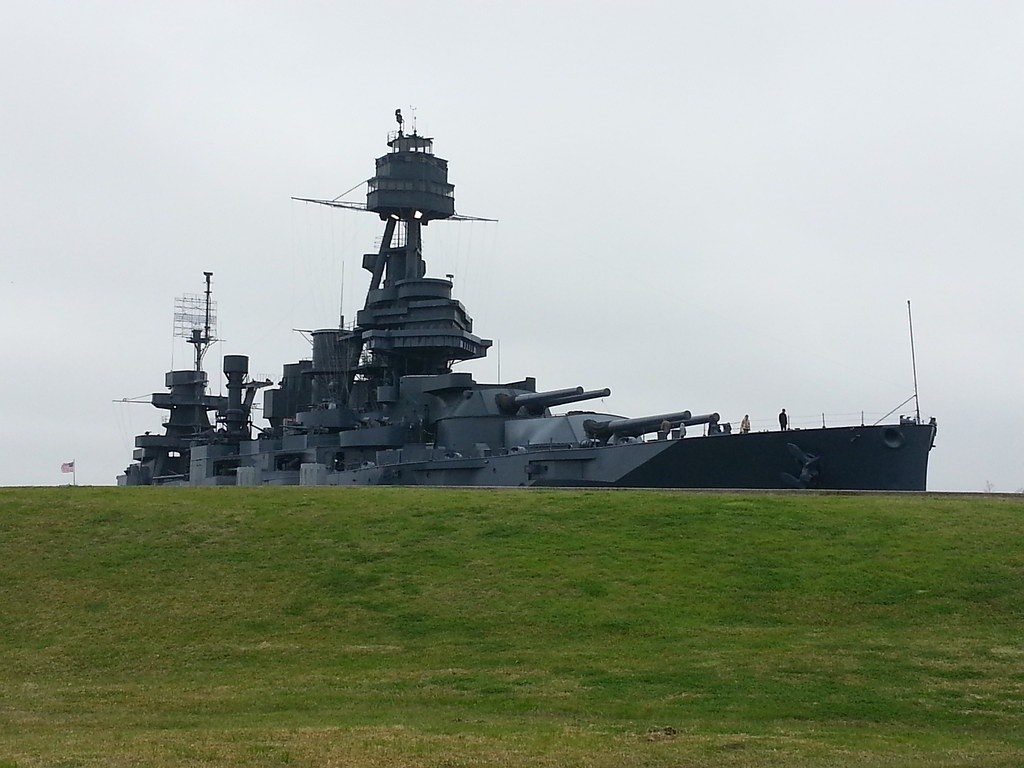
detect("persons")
[779,409,787,431]
[740,415,750,434]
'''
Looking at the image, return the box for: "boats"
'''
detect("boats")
[111,129,939,491]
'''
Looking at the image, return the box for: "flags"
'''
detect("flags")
[61,463,73,473]
